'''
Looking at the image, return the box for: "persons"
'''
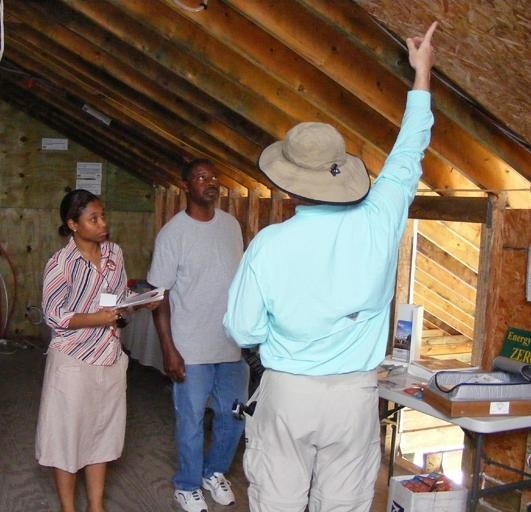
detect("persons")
[220,15,440,511]
[30,187,164,511]
[146,156,251,512]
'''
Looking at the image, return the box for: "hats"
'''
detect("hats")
[257,121,371,205]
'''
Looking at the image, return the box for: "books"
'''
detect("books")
[392,302,483,383]
[113,287,166,310]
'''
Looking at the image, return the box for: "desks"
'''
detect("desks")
[371,377,531,512]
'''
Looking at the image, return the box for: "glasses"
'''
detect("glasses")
[115,312,127,328]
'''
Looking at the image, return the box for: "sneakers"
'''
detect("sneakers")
[174,486,209,511]
[201,471,236,506]
